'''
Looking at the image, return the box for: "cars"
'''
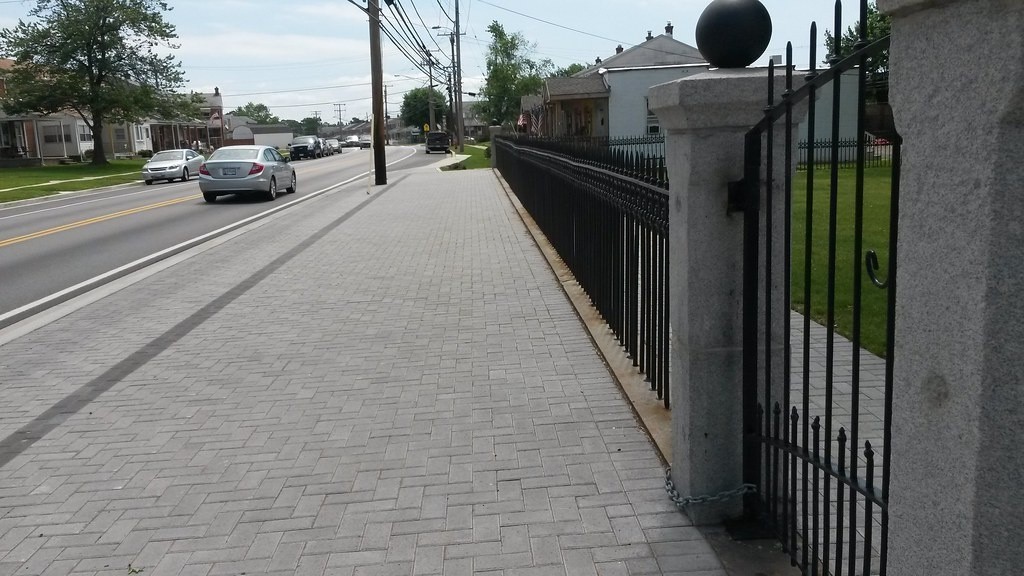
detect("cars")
[198,144,296,202]
[142,148,206,185]
[339,140,347,148]
[317,137,342,157]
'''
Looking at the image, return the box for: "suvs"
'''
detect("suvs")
[360,134,373,150]
[425,131,451,154]
[288,134,323,161]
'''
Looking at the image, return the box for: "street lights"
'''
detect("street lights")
[432,25,459,145]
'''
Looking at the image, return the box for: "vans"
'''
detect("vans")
[346,135,359,147]
